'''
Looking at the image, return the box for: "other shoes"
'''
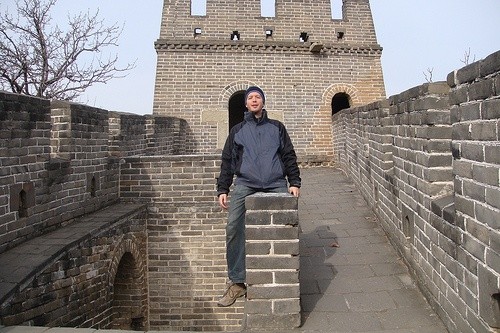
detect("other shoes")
[217,282,247,306]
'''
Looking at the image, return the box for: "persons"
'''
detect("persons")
[217,86,301,307]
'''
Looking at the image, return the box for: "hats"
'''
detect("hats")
[245,85,265,107]
